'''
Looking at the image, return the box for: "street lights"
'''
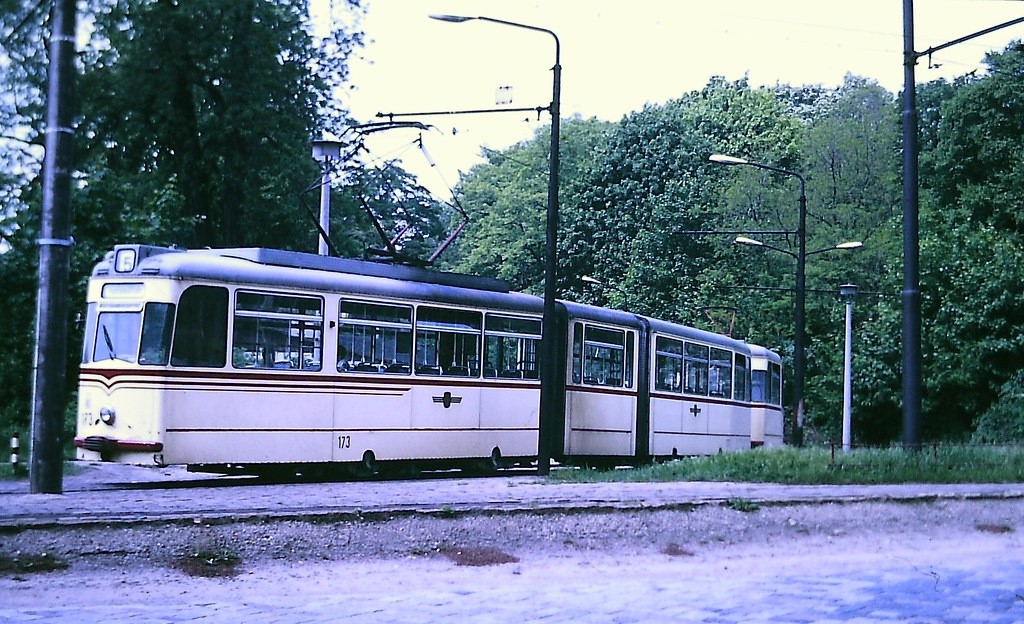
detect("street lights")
[708,151,807,447]
[835,282,862,452]
[580,274,629,311]
[426,12,564,477]
[306,137,351,368]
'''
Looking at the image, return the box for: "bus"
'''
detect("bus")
[71,242,785,477]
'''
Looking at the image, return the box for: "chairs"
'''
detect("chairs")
[273,357,726,398]
